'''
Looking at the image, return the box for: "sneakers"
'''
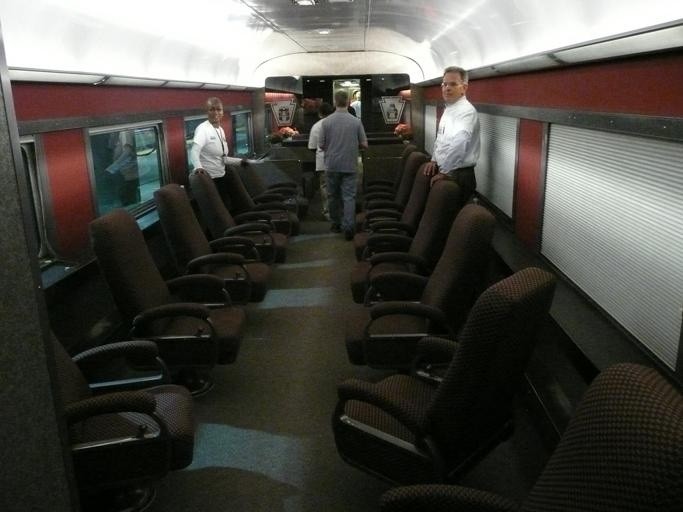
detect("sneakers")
[343,226,354,241]
[330,223,342,233]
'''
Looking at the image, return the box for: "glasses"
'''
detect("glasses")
[441,81,464,89]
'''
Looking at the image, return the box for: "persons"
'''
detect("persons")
[190,97,249,217]
[104,130,140,207]
[292,97,320,133]
[308,102,334,221]
[423,66,481,227]
[318,91,368,242]
